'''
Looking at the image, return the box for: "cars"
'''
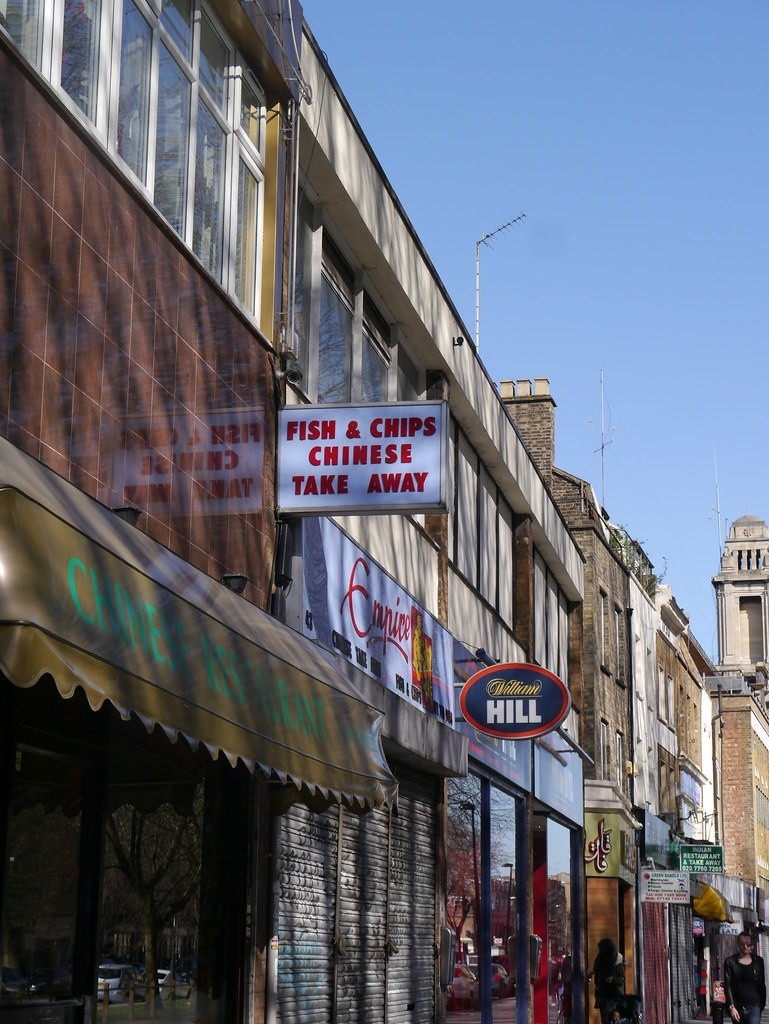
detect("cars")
[1,957,171,1003]
[445,955,510,1011]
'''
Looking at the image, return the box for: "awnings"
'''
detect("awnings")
[691,882,734,924]
[1,435,396,815]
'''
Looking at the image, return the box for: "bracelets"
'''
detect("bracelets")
[728,1006,734,1010]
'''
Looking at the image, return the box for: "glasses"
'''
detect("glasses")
[740,941,753,947]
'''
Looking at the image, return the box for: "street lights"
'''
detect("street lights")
[459,803,479,946]
[500,861,513,951]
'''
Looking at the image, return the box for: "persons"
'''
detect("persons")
[609,1009,633,1024]
[594,938,624,1024]
[549,954,573,1024]
[723,932,766,1024]
